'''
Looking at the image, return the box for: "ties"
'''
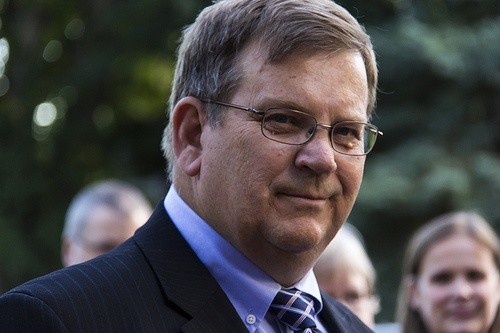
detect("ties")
[270,288,323,333]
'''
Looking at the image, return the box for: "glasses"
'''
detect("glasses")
[196,97,383,156]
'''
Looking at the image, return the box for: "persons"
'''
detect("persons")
[311,222,382,333]
[0,0,380,333]
[60,173,157,267]
[394,210,500,333]
